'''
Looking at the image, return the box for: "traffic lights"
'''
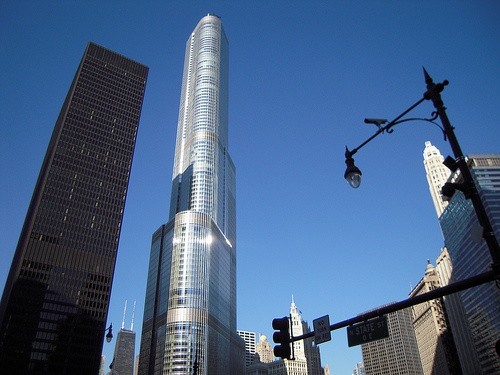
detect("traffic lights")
[271,313,296,361]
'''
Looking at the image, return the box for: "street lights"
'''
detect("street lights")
[343,64,500,265]
